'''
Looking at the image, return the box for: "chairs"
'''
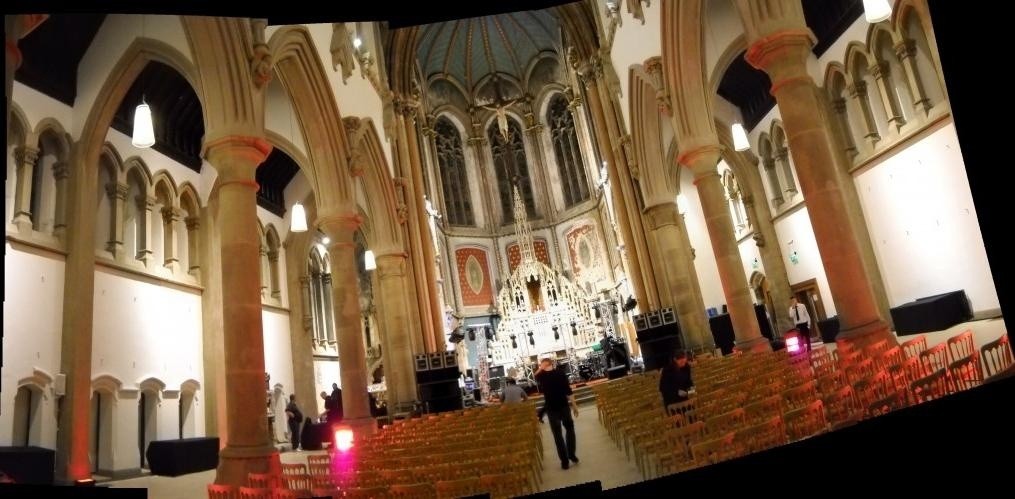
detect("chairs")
[307,433,385,497]
[377,399,544,497]
[776,336,890,443]
[207,464,311,497]
[947,330,1013,392]
[869,336,949,418]
[593,350,789,481]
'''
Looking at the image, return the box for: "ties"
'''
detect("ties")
[795,306,799,321]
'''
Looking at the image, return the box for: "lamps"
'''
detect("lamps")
[73,475,96,486]
[363,210,379,273]
[128,13,158,152]
[705,1,757,153]
[442,293,637,348]
[287,101,311,233]
[857,0,897,26]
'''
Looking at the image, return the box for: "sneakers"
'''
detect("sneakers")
[560,457,570,470]
[567,453,579,464]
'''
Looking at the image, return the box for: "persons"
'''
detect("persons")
[271,382,383,454]
[535,359,584,469]
[500,378,527,404]
[786,298,814,351]
[658,351,699,415]
[537,407,550,421]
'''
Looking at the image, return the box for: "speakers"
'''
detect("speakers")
[606,363,627,380]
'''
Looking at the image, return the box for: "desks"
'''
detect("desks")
[142,432,224,478]
[885,289,978,335]
[0,443,58,484]
[814,312,843,345]
